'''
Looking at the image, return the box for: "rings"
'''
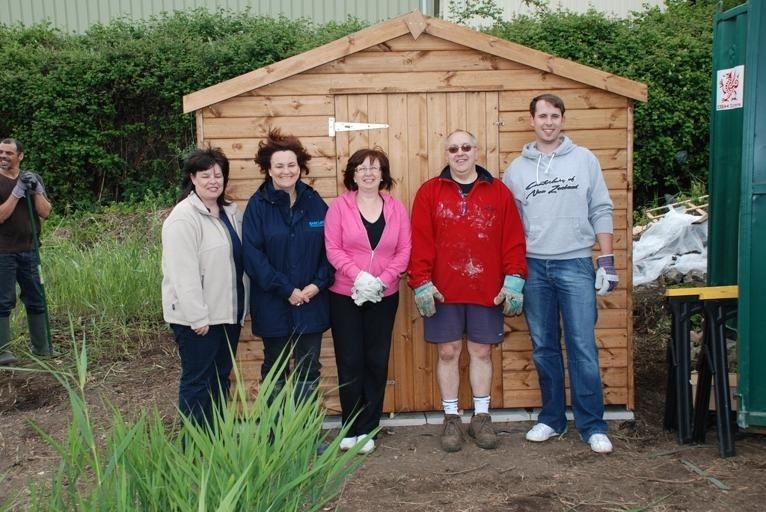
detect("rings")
[295,302,301,307]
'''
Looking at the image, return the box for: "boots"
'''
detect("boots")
[27,311,69,358]
[0,317,18,365]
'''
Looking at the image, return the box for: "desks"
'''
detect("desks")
[665,287,707,444]
[699,285,739,458]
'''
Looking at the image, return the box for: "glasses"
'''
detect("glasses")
[446,144,476,154]
[355,165,381,173]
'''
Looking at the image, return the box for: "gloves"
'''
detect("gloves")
[414,281,444,318]
[12,171,45,198]
[594,254,619,296]
[351,271,387,306]
[493,275,526,317]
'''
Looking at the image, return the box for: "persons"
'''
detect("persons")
[324,148,411,454]
[162,148,250,462]
[1,138,61,366]
[408,130,529,451]
[240,126,336,456]
[502,95,618,455]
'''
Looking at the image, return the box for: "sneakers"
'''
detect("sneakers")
[440,414,465,452]
[356,434,375,454]
[526,423,568,442]
[578,431,613,453]
[340,434,356,450]
[467,411,497,450]
